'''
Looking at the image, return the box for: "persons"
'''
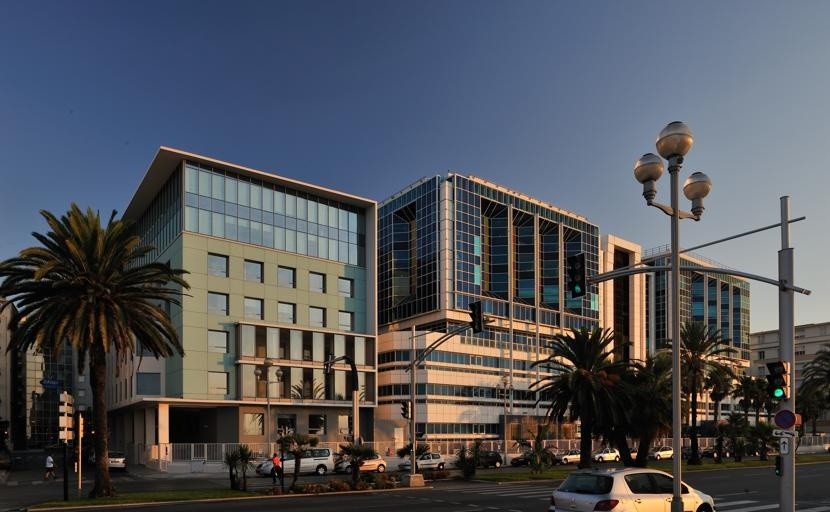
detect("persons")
[271,453,284,485]
[44,452,58,481]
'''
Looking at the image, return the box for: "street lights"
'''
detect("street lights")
[630,118,711,511]
[254,357,283,458]
[496,375,514,466]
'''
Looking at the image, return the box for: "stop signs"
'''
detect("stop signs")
[772,409,797,430]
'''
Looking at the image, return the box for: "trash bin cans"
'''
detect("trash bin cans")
[388,447,394,457]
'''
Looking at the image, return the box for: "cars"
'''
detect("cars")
[548,466,717,512]
[334,454,388,474]
[455,449,502,469]
[397,452,445,471]
[90,450,126,470]
[510,444,734,467]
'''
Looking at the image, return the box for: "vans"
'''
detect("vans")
[255,448,334,477]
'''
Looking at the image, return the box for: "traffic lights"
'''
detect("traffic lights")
[400,399,412,420]
[566,252,588,299]
[468,299,484,334]
[765,360,790,401]
